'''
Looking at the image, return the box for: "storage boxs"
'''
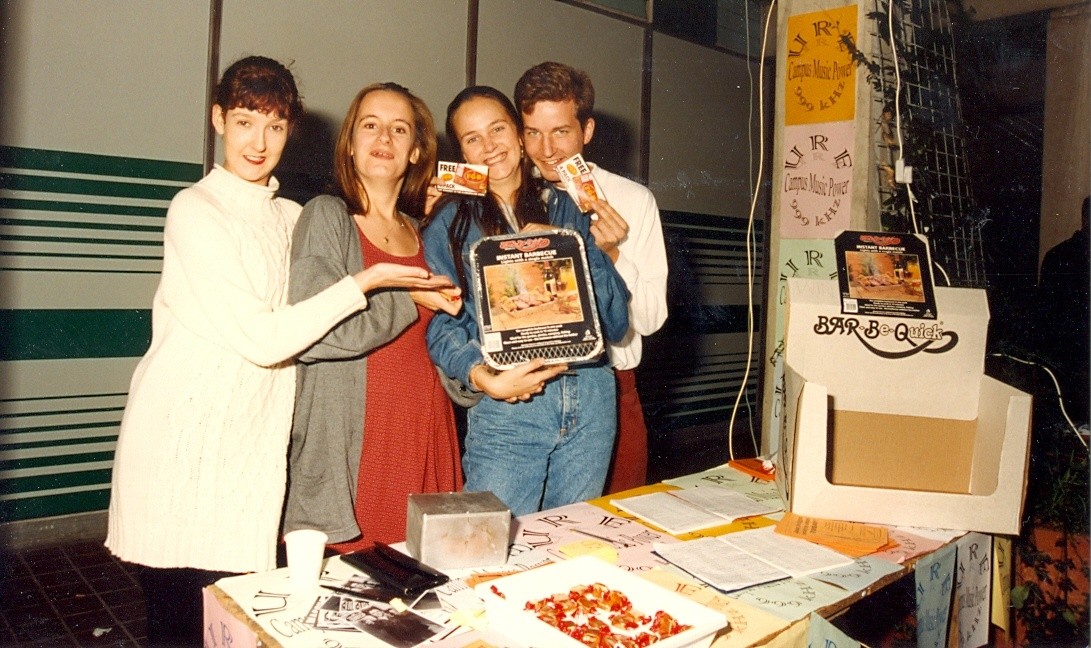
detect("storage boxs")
[406,491,513,566]
[777,279,1033,535]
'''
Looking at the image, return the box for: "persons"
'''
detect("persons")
[424,60,669,495]
[1040,198,1090,289]
[103,57,453,648]
[363,605,437,645]
[347,580,419,602]
[428,87,633,518]
[280,82,466,555]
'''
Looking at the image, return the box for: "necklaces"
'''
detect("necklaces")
[384,225,398,244]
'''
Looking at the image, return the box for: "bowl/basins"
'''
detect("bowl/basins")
[475,555,728,648]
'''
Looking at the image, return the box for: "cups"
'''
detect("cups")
[284,529,329,585]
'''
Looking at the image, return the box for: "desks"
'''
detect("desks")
[204,456,1011,648]
